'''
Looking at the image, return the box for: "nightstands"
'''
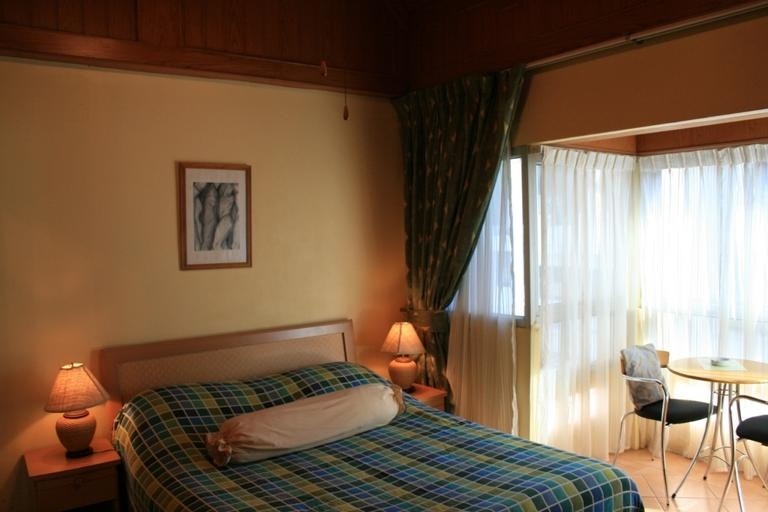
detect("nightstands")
[23,441,121,512]
[410,382,449,411]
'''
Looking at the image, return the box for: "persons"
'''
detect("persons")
[198,182,238,250]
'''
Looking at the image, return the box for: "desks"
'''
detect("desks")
[670,354,768,512]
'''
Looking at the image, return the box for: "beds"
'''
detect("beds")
[95,319,647,512]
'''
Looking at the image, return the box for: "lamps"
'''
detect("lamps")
[381,321,426,391]
[42,363,110,460]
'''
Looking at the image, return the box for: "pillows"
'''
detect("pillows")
[206,384,408,462]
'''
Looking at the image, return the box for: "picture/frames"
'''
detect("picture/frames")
[176,162,254,271]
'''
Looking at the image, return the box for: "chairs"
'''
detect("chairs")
[725,394,768,512]
[614,344,722,507]
